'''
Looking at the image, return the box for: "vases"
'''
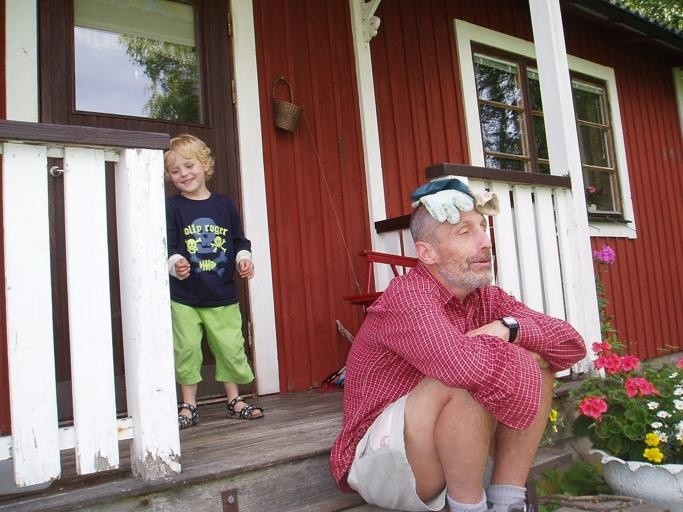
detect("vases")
[586,446,683,512]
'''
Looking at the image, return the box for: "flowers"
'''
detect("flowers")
[566,340,683,465]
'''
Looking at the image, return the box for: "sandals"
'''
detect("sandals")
[178,402,200,430]
[226,396,264,420]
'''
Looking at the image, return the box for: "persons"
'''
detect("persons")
[330,202,586,512]
[165,135,265,430]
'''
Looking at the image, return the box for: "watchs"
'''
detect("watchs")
[496,316,518,343]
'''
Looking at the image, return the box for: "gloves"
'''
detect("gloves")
[411,176,500,224]
